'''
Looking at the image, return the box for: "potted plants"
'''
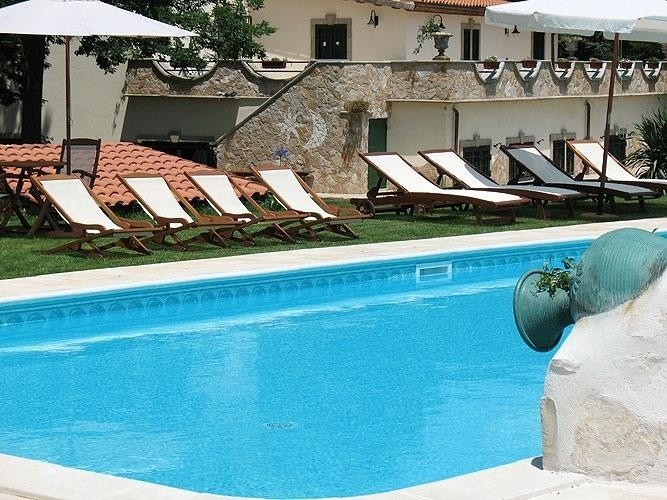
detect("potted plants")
[484,57,659,68]
[411,16,453,60]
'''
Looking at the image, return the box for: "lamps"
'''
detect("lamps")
[433,14,446,29]
[366,9,378,27]
[504,24,520,37]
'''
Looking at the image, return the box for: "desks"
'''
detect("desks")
[0,161,63,232]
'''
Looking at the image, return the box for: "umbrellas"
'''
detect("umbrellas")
[0,0,201,174]
[481,0,665,214]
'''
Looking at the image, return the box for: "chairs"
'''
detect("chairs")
[417,148,588,223]
[501,144,658,215]
[249,161,375,243]
[565,140,667,212]
[184,170,312,243]
[114,173,245,251]
[31,138,103,225]
[31,172,167,259]
[356,152,534,228]
